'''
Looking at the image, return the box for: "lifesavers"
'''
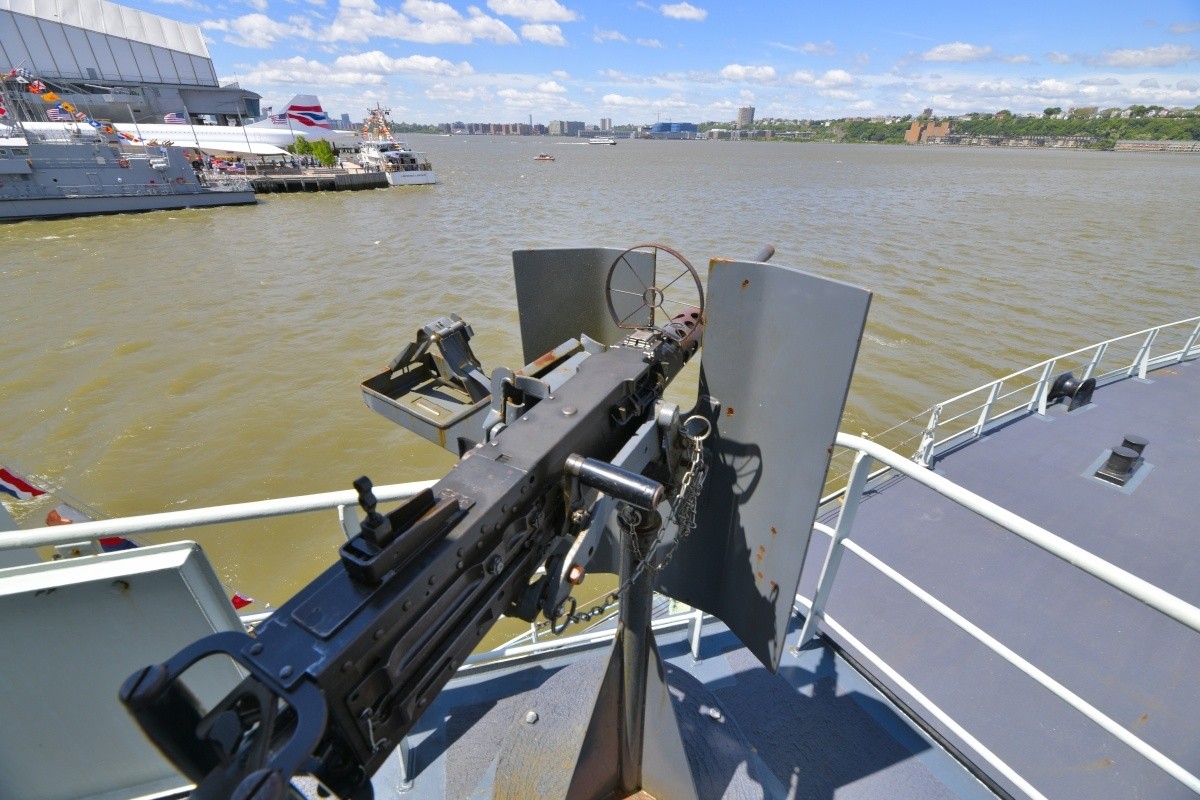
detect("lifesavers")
[176,176,185,184]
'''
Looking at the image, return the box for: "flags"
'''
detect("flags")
[164,112,186,123]
[0,68,145,143]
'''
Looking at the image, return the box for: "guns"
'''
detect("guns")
[116,238,786,799]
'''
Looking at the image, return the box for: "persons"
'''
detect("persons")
[183,151,243,187]
[289,155,343,168]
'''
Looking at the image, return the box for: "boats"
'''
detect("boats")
[534,154,557,161]
[356,99,437,186]
[589,135,617,145]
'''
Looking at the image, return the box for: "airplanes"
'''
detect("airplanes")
[1,93,363,155]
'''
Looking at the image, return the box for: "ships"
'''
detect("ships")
[0,113,256,221]
